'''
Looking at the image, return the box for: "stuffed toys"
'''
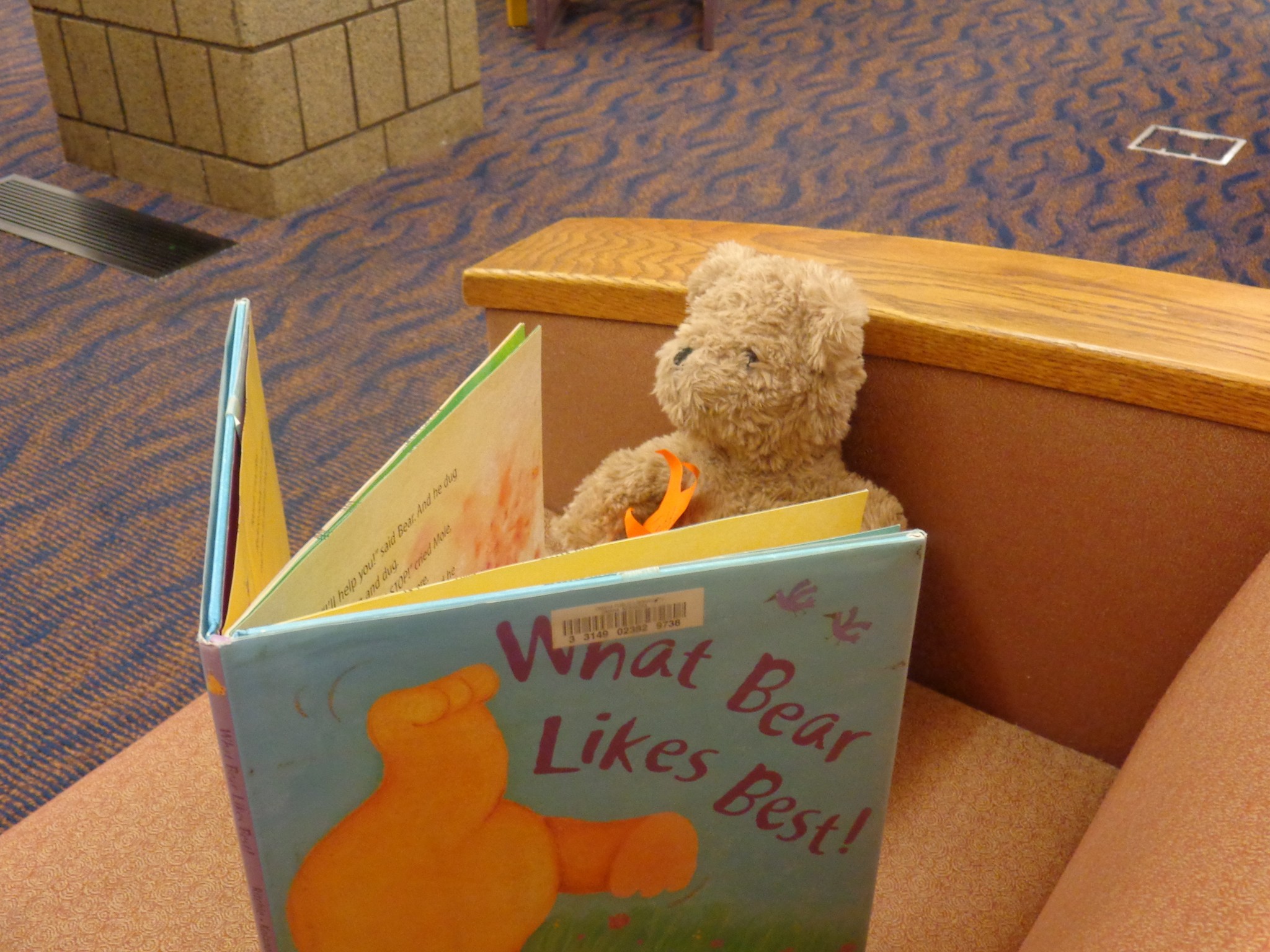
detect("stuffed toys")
[553,243,908,551]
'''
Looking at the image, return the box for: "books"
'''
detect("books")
[197,299,924,951]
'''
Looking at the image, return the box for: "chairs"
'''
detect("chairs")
[0,218,1269,952]
